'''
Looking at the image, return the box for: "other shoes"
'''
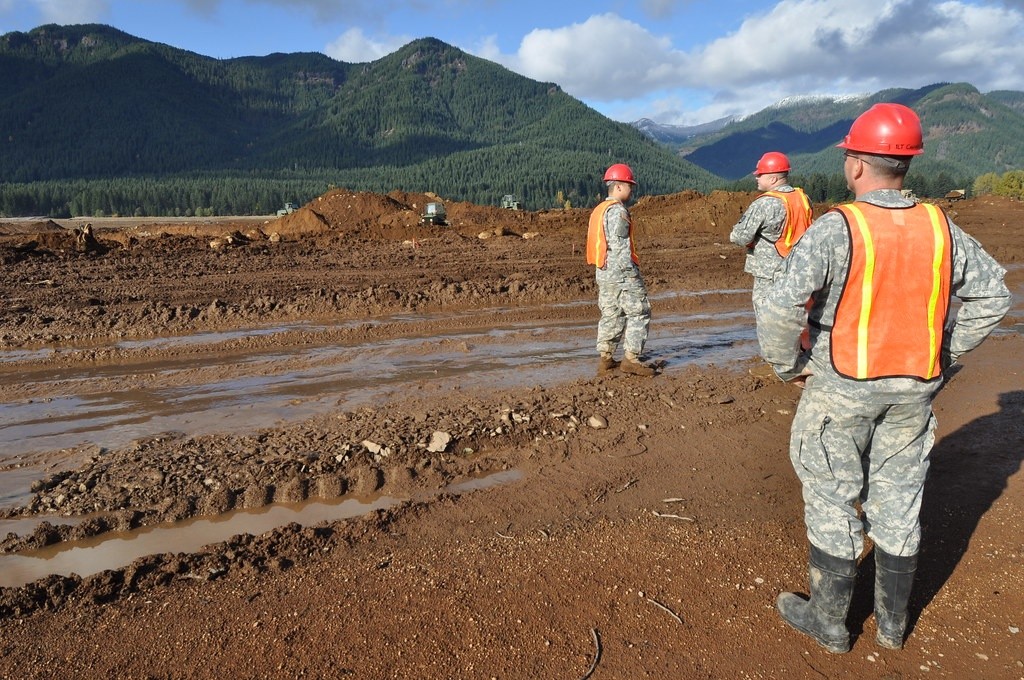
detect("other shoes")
[600,356,654,376]
[749,363,781,380]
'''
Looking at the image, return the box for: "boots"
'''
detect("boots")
[777,543,919,655]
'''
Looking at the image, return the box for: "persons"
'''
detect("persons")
[585,164,657,378]
[728,152,815,390]
[756,102,1011,654]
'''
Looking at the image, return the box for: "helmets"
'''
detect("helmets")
[835,102,924,155]
[603,164,637,185]
[753,152,791,175]
